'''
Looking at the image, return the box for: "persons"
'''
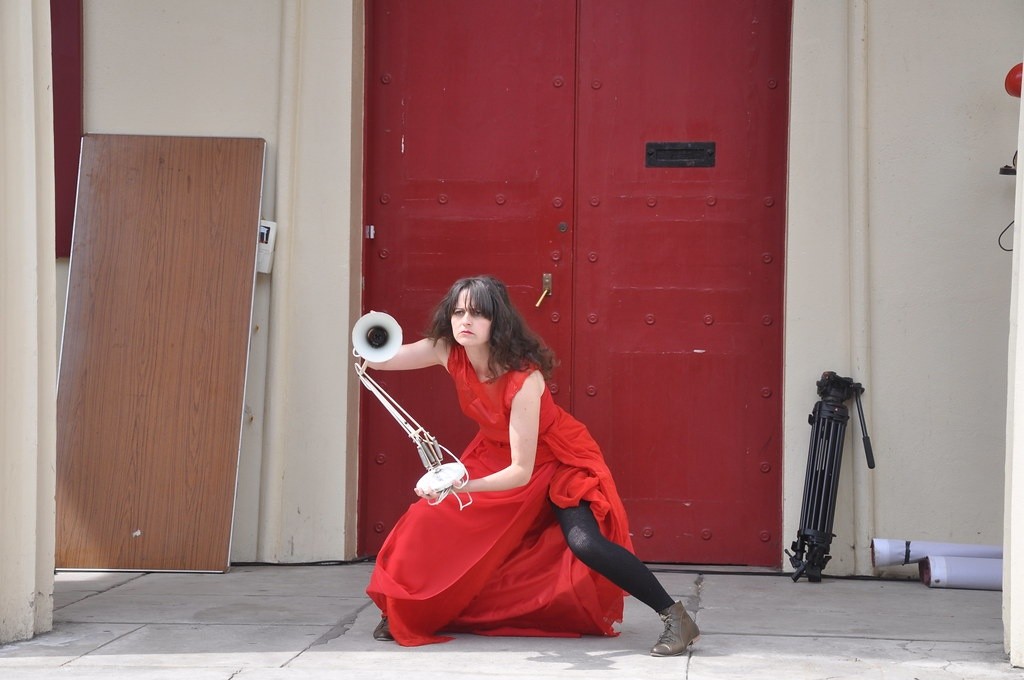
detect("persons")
[366,274,701,656]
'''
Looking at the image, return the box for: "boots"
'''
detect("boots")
[650,600,700,657]
[373,616,394,641]
